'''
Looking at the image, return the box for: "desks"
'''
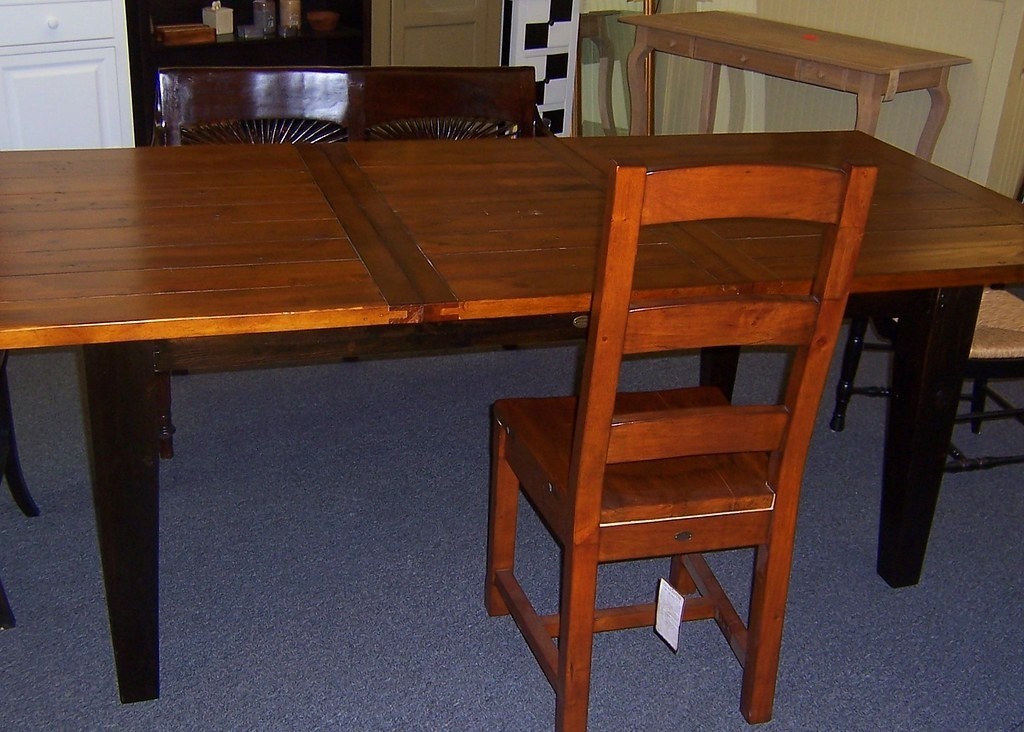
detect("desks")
[616,10,972,163]
[572,10,644,136]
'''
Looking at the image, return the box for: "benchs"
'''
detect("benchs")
[127,66,558,460]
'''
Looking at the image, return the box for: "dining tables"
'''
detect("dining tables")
[0,130,1024,705]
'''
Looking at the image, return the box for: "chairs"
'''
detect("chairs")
[0,349,41,630]
[829,170,1024,474]
[484,165,878,732]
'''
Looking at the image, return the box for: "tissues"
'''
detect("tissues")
[201,1,233,35]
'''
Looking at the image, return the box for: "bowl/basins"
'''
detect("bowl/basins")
[307,11,341,31]
[278,24,298,38]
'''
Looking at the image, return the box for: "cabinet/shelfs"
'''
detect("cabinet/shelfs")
[0,0,135,152]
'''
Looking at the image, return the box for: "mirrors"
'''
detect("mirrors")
[572,0,655,137]
